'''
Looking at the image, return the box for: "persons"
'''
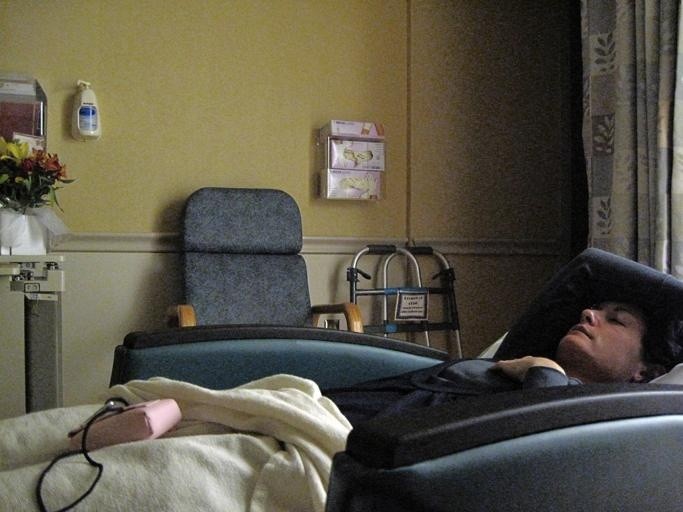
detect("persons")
[298,243,681,452]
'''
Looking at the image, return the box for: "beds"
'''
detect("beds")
[0,323,683,512]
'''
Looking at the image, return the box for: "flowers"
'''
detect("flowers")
[0,133,75,215]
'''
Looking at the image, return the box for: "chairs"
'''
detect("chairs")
[165,187,366,335]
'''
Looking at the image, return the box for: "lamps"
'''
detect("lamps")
[0,212,47,255]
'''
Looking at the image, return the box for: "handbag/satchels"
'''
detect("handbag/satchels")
[68,397,182,453]
